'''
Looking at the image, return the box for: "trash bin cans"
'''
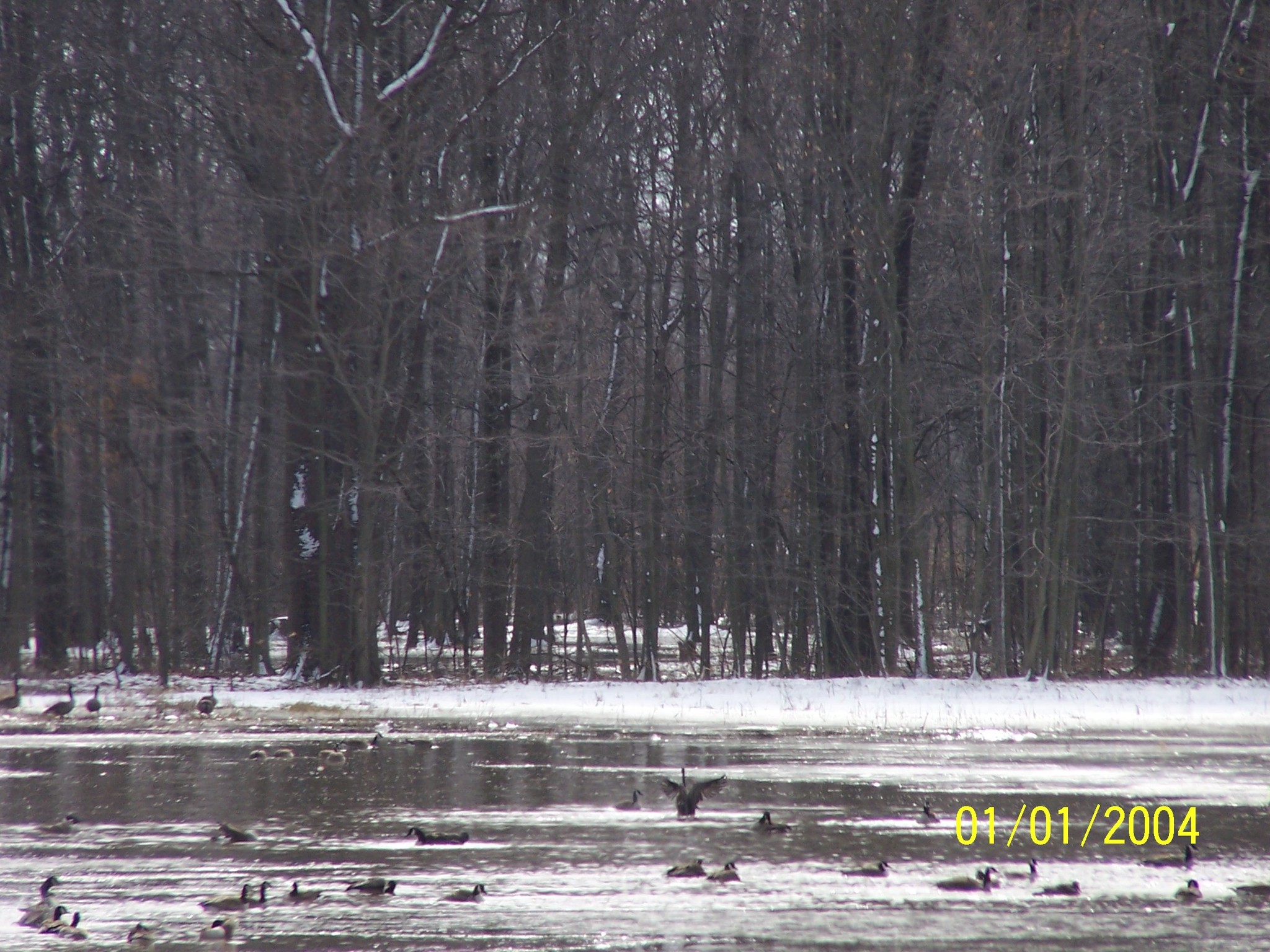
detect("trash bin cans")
[678,641,696,661]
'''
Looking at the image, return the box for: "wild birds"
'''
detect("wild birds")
[196,685,217,718]
[32,813,80,836]
[0,671,23,715]
[11,876,88,941]
[41,684,76,722]
[84,685,102,718]
[476,720,521,729]
[614,766,792,882]
[840,800,1270,902]
[126,733,488,943]
